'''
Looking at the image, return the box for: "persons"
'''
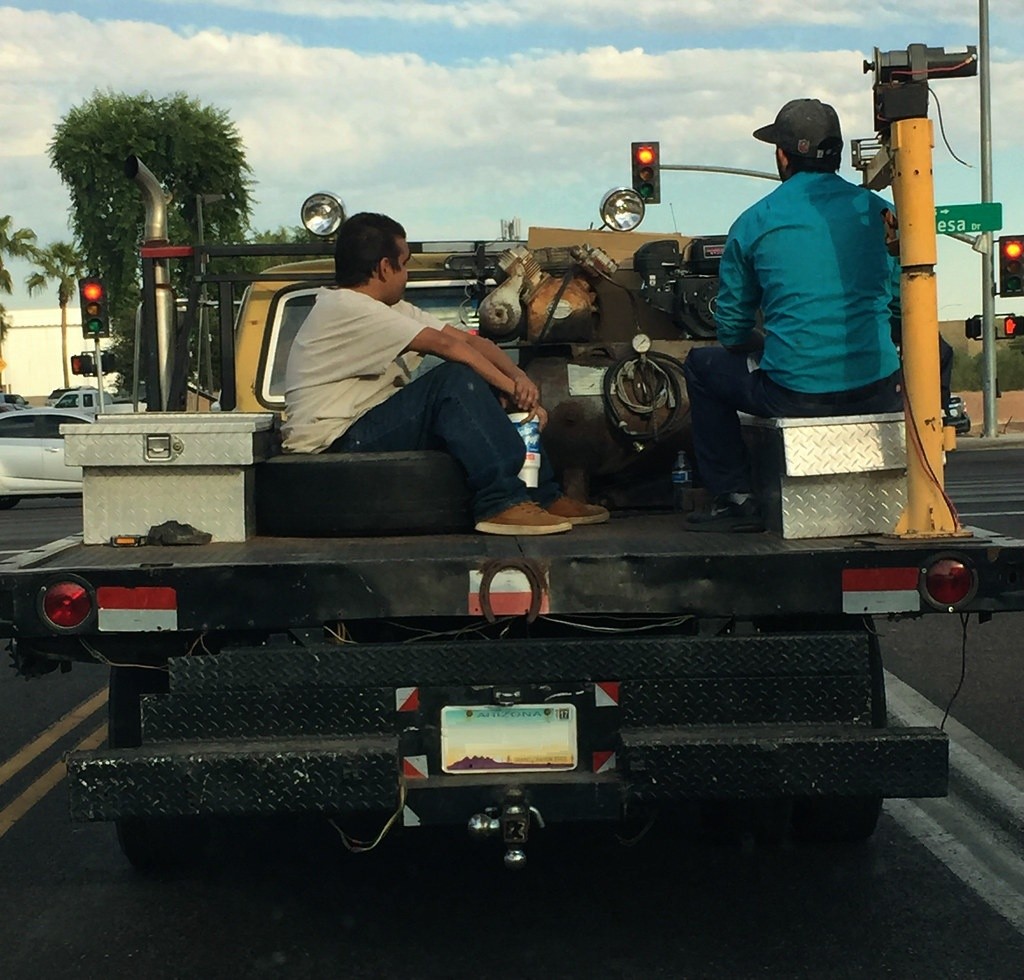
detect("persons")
[678,98,956,535]
[279,212,609,534]
[634,167,658,201]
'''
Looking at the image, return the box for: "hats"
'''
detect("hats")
[752,98,843,159]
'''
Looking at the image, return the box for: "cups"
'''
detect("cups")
[507,412,541,488]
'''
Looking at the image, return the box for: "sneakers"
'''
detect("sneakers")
[475,501,572,535]
[544,493,610,524]
[683,487,765,532]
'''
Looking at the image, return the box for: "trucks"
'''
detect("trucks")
[0,187,1024,893]
[52,390,148,420]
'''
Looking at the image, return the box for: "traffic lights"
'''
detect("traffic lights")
[965,317,982,339]
[630,141,662,206]
[998,234,1024,297]
[71,354,91,378]
[1003,315,1024,336]
[77,275,112,341]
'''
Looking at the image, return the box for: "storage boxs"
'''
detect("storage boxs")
[756,470,908,540]
[739,409,947,476]
[82,466,255,545]
[59,417,270,465]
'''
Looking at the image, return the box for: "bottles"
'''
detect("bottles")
[672,451,695,512]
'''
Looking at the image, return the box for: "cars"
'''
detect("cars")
[947,394,972,433]
[45,386,82,408]
[0,393,35,414]
[0,409,99,510]
